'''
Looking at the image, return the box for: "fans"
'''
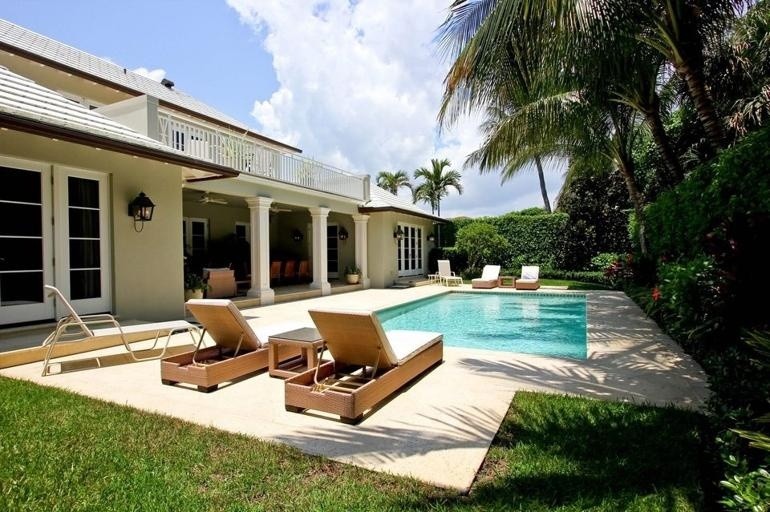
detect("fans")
[268,203,294,214]
[195,189,230,207]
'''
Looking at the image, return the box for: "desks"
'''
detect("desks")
[428,274,440,286]
[264,326,331,381]
[497,276,518,289]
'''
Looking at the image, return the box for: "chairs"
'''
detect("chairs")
[270,260,283,283]
[281,305,446,427]
[281,260,296,279]
[158,295,328,396]
[515,265,541,291]
[295,259,309,279]
[38,280,208,380]
[435,258,464,288]
[471,263,505,290]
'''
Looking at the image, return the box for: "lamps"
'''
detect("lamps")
[126,188,156,233]
[337,226,349,241]
[393,227,405,241]
[426,231,437,242]
[292,229,303,241]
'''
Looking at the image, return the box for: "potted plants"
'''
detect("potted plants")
[185,272,213,302]
[345,264,361,285]
